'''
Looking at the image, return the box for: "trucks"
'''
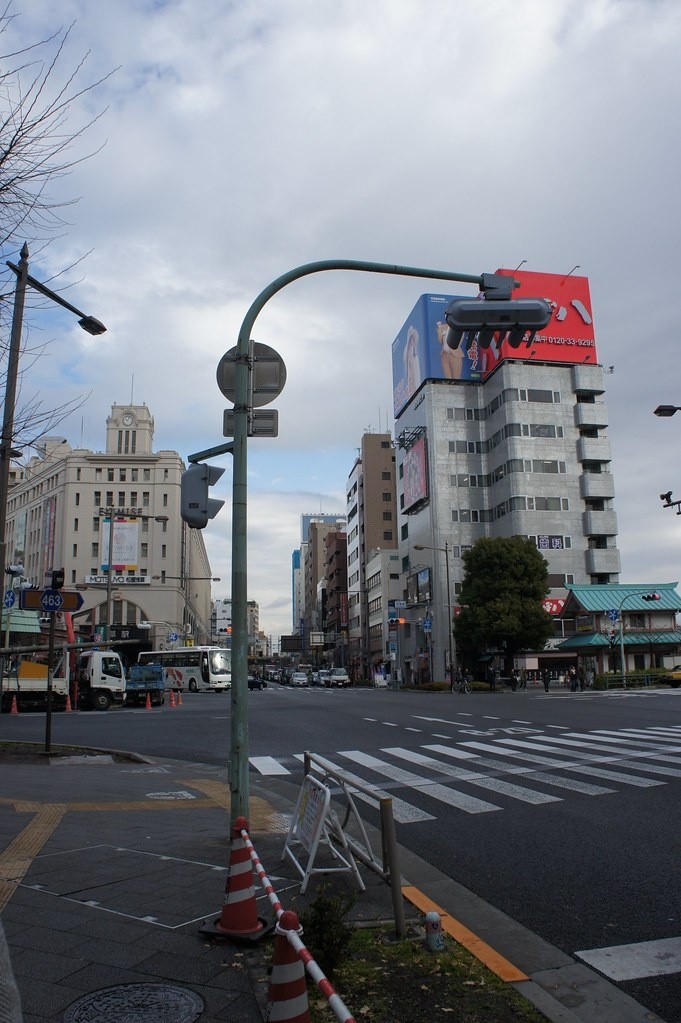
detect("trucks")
[0,647,127,711]
[123,663,167,707]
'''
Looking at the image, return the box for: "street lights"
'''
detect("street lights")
[0,240,107,708]
[334,589,371,680]
[152,574,223,647]
[412,538,470,691]
[104,505,171,655]
[322,631,345,668]
[207,617,232,646]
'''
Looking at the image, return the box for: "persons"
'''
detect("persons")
[487,667,496,693]
[543,669,550,692]
[519,666,528,691]
[436,307,465,379]
[403,326,421,395]
[454,667,470,684]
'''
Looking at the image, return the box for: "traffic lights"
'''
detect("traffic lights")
[38,617,51,624]
[4,564,23,577]
[21,583,40,591]
[219,627,231,633]
[388,617,405,625]
[440,294,553,351]
[178,462,226,530]
[642,593,661,601]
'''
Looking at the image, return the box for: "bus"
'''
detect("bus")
[136,646,232,693]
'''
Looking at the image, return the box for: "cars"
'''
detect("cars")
[247,675,267,691]
[251,662,351,689]
[659,664,681,688]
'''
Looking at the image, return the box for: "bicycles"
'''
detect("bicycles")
[451,674,473,694]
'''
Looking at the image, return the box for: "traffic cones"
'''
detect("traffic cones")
[10,694,20,715]
[144,692,153,710]
[168,689,177,708]
[262,909,313,1023]
[64,692,73,713]
[176,691,183,705]
[198,816,277,946]
